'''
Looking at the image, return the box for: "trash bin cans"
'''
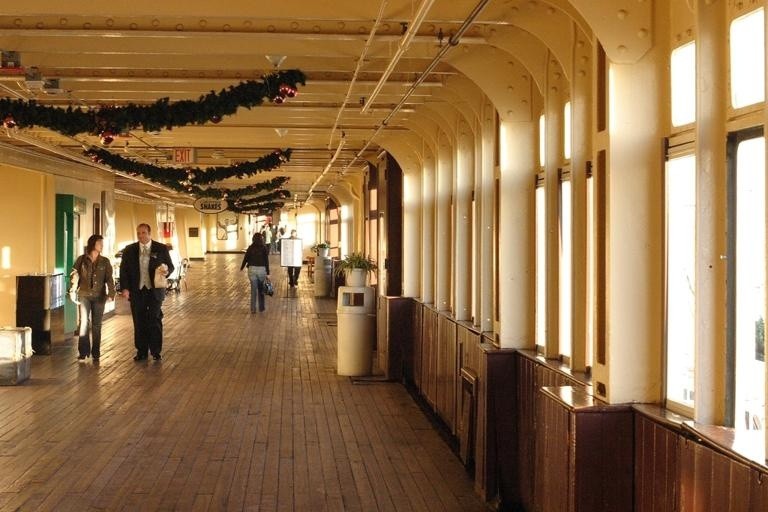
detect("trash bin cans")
[336,287,376,375]
[314,257,333,297]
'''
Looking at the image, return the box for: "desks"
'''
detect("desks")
[306,256,314,278]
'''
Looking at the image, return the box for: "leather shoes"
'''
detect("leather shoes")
[78,352,89,359]
[151,353,160,360]
[133,354,148,360]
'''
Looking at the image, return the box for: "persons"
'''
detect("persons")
[286,229,300,287]
[240,232,269,314]
[261,223,285,253]
[165,241,182,281]
[68,235,115,359]
[121,224,174,361]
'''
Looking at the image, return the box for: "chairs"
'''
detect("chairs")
[165,258,188,296]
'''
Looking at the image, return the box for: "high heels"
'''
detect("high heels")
[93,353,99,360]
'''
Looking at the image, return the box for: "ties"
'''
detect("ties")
[142,246,147,254]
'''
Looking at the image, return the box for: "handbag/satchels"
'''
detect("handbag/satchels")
[260,279,272,295]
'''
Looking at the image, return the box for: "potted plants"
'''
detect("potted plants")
[318,243,330,257]
[334,252,377,286]
